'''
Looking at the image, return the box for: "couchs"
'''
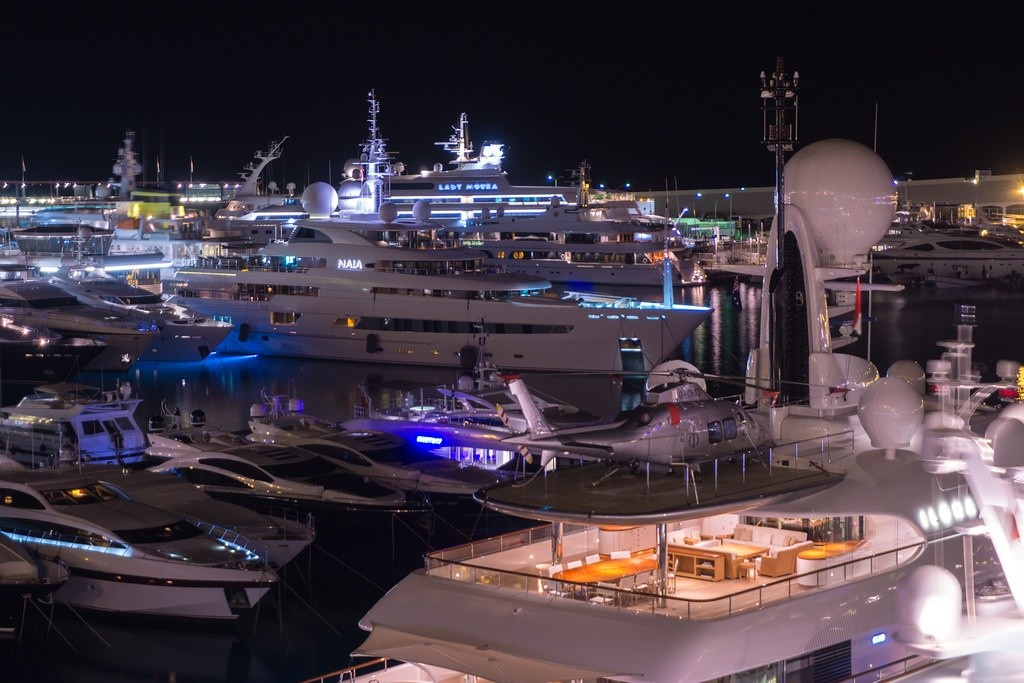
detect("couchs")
[667,525,721,547]
[723,524,807,556]
[757,541,815,578]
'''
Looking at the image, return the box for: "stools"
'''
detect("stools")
[739,563,756,581]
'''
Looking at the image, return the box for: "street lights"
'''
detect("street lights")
[692,192,701,238]
[548,175,557,187]
[730,187,746,220]
[714,193,729,227]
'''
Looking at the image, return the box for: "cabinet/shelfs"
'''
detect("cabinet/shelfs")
[668,547,725,581]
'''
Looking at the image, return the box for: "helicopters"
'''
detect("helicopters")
[495,367,852,485]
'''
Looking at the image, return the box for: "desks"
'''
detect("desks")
[552,557,658,600]
[536,564,552,577]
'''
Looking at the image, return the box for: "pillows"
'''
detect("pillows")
[740,530,753,541]
[772,533,785,546]
[752,529,763,543]
[784,535,791,546]
[790,537,799,546]
[691,531,701,541]
[673,536,686,544]
[734,528,746,540]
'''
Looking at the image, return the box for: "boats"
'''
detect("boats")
[1,85,1024,682]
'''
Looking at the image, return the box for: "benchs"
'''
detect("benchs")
[668,543,746,580]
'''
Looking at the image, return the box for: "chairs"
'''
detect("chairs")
[548,551,679,608]
